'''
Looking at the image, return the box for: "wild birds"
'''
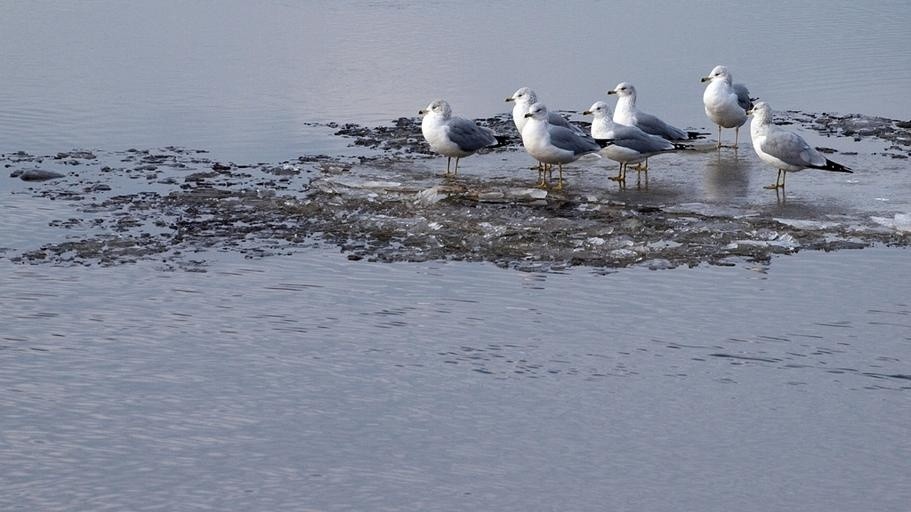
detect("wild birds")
[418,97,500,177]
[503,86,587,171]
[745,101,853,190]
[698,64,754,149]
[522,101,601,190]
[582,100,676,182]
[606,81,689,171]
